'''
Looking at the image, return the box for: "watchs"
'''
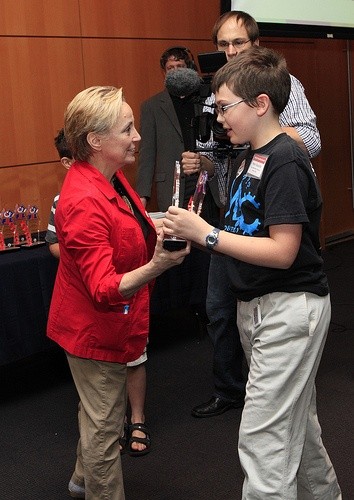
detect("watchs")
[206,228,220,250]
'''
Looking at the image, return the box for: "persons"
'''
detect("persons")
[181,12,322,417]
[135,47,211,345]
[44,128,150,454]
[163,47,344,500]
[47,85,191,500]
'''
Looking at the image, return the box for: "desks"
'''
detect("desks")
[0,230,63,380]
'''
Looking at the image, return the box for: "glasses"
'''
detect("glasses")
[214,91,271,116]
[217,38,252,51]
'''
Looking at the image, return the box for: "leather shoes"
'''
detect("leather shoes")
[192,395,243,417]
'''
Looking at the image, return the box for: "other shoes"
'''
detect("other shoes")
[68,481,86,498]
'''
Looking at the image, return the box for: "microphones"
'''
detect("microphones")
[164,68,204,97]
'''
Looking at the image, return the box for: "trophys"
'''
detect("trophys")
[157,160,206,241]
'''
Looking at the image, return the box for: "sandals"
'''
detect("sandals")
[129,424,149,457]
[117,424,128,455]
